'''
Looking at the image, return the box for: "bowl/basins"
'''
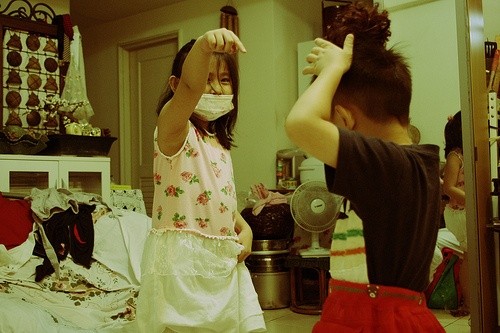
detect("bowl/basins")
[254,240,277,251]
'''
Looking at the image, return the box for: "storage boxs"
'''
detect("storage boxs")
[34,134,117,157]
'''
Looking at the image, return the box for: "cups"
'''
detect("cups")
[281,181,297,190]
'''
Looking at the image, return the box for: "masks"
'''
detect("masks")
[191,92,235,122]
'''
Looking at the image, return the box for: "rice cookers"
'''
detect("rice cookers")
[297,157,326,191]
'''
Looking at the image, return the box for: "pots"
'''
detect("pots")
[250,272,290,310]
[245,255,284,272]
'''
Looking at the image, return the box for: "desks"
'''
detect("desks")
[287,256,330,315]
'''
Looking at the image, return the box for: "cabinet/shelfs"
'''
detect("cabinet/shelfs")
[0,154,111,201]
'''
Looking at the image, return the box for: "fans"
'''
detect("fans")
[290,180,340,259]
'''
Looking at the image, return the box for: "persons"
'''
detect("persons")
[286,0,446,333]
[135,29,266,333]
[444,111,473,312]
